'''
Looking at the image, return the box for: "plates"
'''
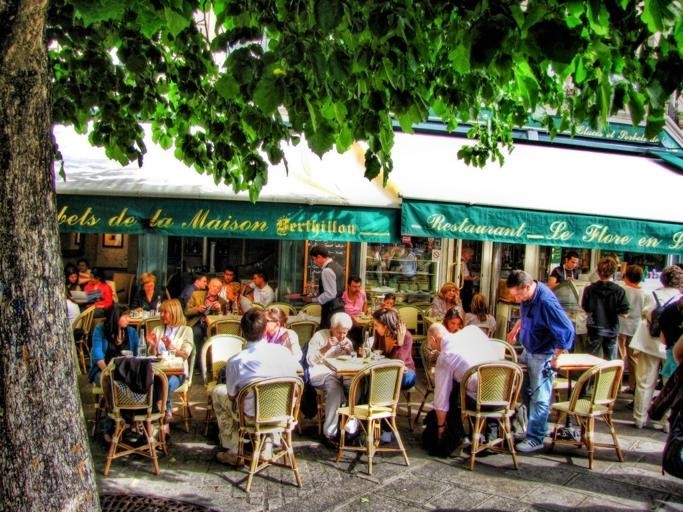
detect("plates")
[371,355,384,360]
[356,314,372,320]
[130,317,140,319]
[337,355,352,361]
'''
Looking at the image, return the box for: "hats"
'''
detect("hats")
[141,273,155,285]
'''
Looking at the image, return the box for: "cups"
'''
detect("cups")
[132,311,137,318]
[169,349,176,360]
[218,311,238,320]
[356,348,363,357]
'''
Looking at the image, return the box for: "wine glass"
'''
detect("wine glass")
[305,282,318,296]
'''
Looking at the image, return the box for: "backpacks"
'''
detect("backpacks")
[647,291,681,336]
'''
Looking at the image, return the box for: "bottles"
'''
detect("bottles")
[155,296,161,313]
[642,266,648,283]
[137,328,147,358]
[362,331,370,364]
[652,269,655,278]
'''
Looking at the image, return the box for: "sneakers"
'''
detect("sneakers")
[217,452,244,467]
[516,438,543,452]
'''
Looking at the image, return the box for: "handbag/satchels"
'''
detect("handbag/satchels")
[300,380,317,420]
[196,420,218,440]
[511,401,529,435]
[422,406,464,458]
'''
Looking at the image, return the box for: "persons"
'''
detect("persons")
[59,244,683,480]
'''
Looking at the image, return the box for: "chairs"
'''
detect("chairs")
[101,367,172,483]
[552,360,630,471]
[224,374,306,495]
[71,272,494,438]
[458,362,522,471]
[338,359,418,477]
